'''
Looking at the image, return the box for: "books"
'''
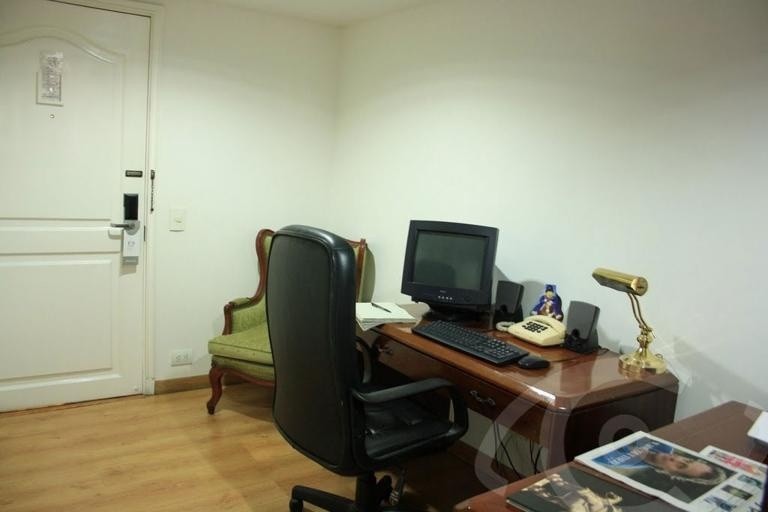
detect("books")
[506,430,768,512]
[355,301,417,332]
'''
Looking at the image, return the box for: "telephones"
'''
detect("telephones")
[496,316,567,348]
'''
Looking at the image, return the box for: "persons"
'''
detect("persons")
[593,437,727,486]
[526,473,622,512]
[531,283,565,320]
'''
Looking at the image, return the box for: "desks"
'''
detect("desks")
[455,400,767,512]
[355,302,679,469]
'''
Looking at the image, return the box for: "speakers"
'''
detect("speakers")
[563,300,601,355]
[491,281,525,331]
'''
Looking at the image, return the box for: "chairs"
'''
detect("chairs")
[207,229,367,415]
[265,225,469,512]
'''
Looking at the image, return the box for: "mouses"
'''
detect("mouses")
[517,355,551,370]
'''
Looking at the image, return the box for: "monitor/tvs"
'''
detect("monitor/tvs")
[400,219,500,323]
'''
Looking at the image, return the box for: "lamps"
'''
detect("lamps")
[593,267,667,373]
[748,411,768,512]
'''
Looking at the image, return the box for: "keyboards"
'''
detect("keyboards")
[411,316,531,366]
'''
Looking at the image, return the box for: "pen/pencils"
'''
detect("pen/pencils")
[372,302,391,313]
